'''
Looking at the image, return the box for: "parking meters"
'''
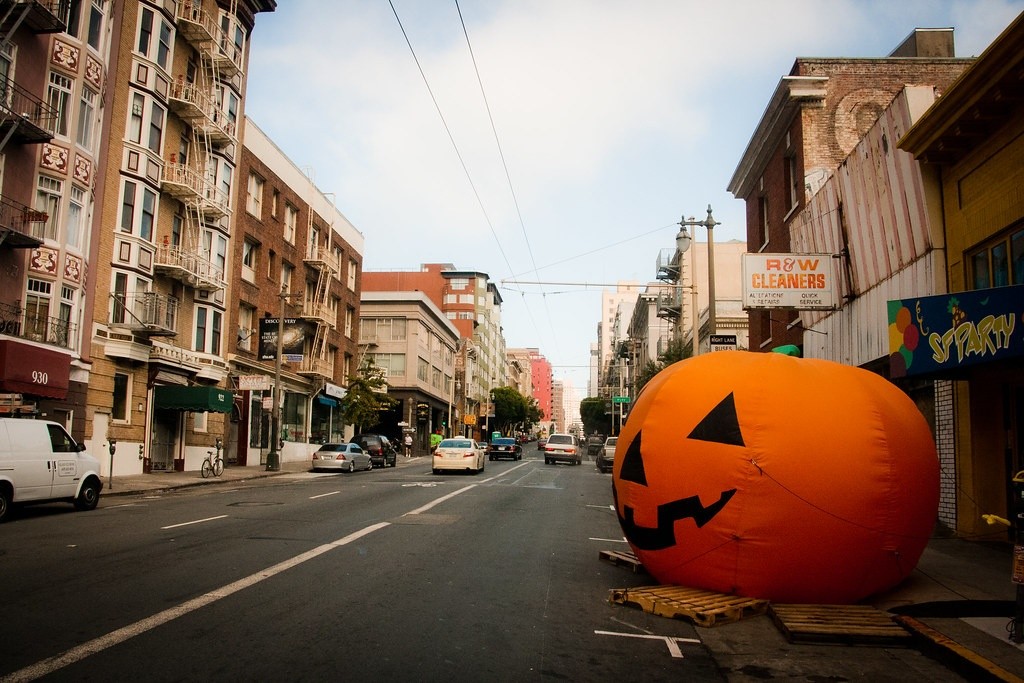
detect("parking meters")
[109,438,116,489]
[322,436,326,446]
[216,437,222,476]
[280,437,284,471]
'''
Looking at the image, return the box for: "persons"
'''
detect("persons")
[405,434,412,458]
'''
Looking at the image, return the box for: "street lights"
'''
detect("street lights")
[486,393,495,441]
[266,290,304,471]
[448,379,461,439]
[676,219,716,336]
[604,390,614,436]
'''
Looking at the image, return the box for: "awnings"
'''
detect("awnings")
[155,386,234,414]
[0,340,72,400]
[317,394,337,406]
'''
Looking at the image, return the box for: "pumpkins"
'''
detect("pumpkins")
[611,345,939,602]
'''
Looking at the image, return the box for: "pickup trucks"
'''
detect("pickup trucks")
[596,436,619,472]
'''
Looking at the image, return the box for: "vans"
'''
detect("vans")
[0,418,105,523]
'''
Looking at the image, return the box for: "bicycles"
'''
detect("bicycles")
[201,451,225,478]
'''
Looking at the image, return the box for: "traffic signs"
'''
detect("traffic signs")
[402,427,417,433]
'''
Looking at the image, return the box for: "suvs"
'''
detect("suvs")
[350,435,397,468]
[544,434,583,464]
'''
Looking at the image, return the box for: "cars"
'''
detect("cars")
[312,442,374,473]
[538,439,547,450]
[490,432,537,461]
[477,442,491,455]
[433,436,485,474]
